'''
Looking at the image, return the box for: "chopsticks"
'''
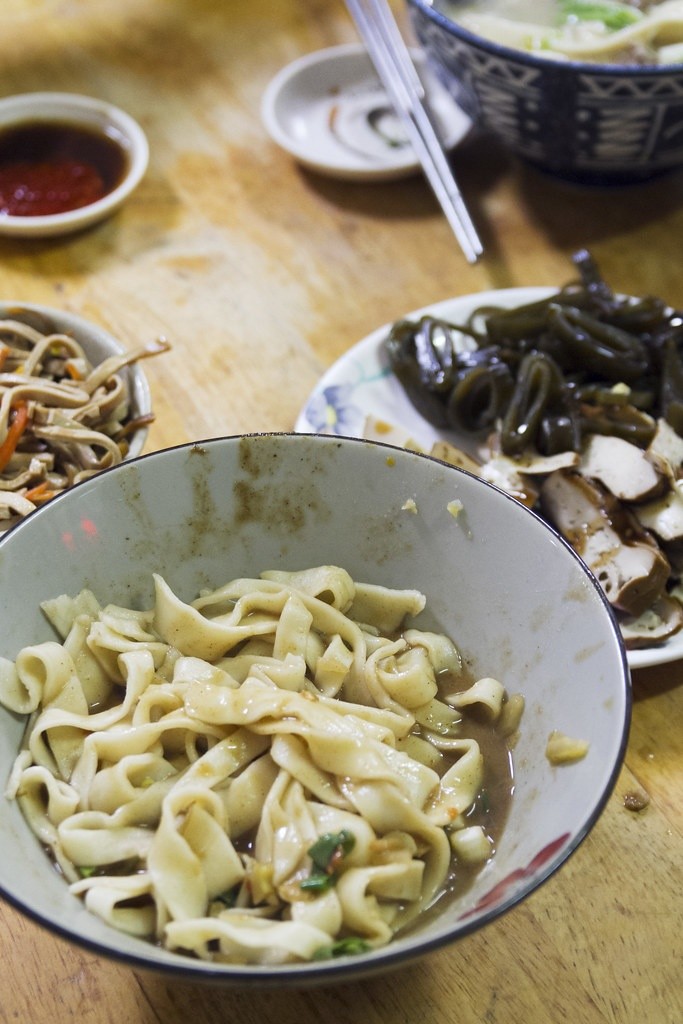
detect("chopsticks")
[344,2,483,264]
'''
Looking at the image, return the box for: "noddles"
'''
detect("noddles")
[1,563,588,962]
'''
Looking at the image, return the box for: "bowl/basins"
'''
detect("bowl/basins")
[1,431,630,992]
[406,3,682,184]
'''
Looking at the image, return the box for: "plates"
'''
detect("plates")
[0,90,149,235]
[291,286,683,669]
[261,39,474,185]
[1,305,155,540]
